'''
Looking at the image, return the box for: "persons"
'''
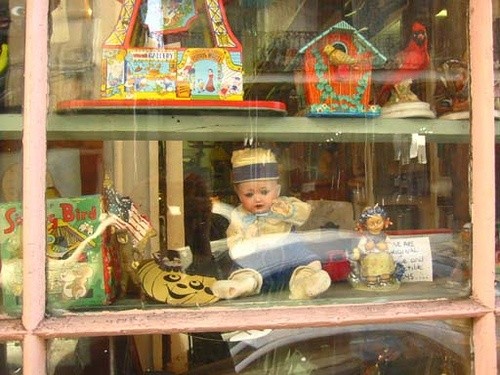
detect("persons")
[196,78,205,93]
[50,172,256,375]
[346,205,398,287]
[212,146,330,298]
[205,68,216,91]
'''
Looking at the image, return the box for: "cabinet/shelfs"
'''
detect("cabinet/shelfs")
[0,0,499,375]
